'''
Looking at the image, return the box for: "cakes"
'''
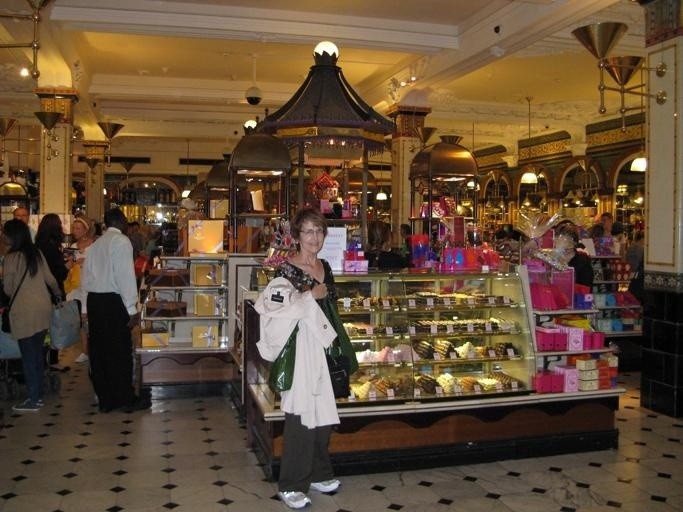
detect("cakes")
[336,290,511,309]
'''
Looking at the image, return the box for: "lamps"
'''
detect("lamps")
[181,142,191,197]
[628,58,647,171]
[243,119,257,133]
[376,153,386,199]
[466,123,480,190]
[312,41,339,65]
[520,97,538,183]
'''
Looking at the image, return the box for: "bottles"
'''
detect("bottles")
[267,267,275,281]
[258,268,267,298]
[346,231,365,261]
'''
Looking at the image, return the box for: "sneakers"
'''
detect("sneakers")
[280,489,312,509]
[25,398,43,406]
[12,404,41,410]
[310,479,340,494]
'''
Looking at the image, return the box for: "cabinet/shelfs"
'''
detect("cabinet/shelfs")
[245,273,626,481]
[135,254,241,387]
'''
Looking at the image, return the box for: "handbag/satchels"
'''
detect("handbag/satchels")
[50,296,81,349]
[324,345,349,398]
[2,252,33,331]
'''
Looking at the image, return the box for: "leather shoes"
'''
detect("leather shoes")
[100,401,121,412]
[122,398,151,410]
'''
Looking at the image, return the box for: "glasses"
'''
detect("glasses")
[299,228,323,235]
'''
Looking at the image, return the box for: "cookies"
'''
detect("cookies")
[348,371,525,399]
[342,317,499,335]
[361,339,519,364]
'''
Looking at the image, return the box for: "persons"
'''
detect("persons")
[35,214,71,373]
[81,208,152,415]
[0,219,63,412]
[556,226,593,290]
[66,216,95,363]
[124,220,143,255]
[398,223,416,259]
[590,212,648,249]
[10,207,38,245]
[367,220,403,269]
[253,207,359,509]
